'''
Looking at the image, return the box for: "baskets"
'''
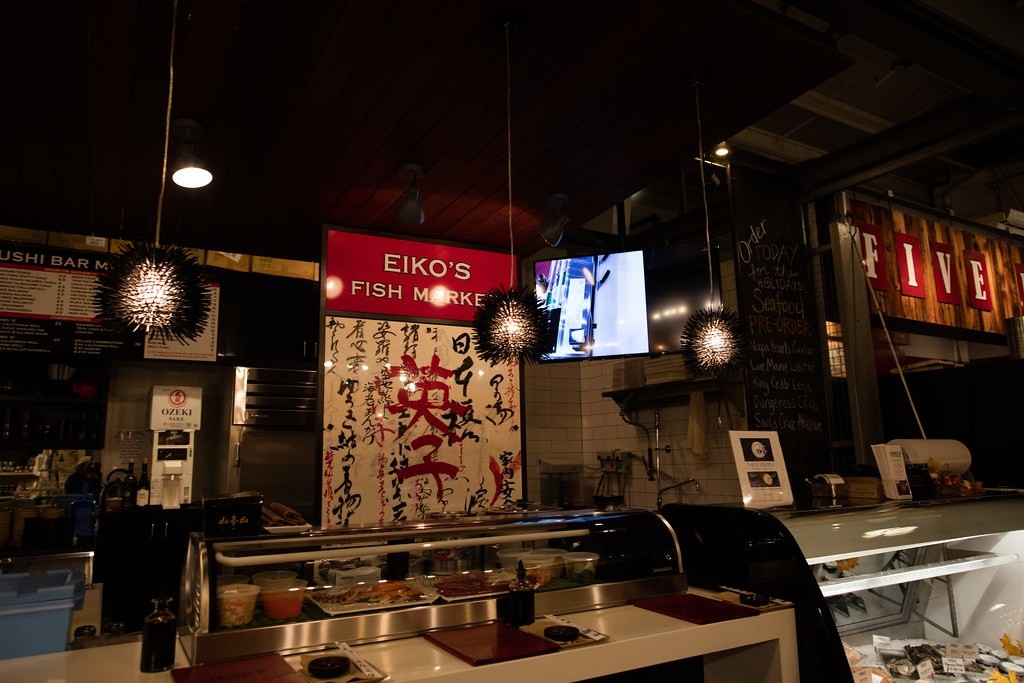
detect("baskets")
[0,507,65,549]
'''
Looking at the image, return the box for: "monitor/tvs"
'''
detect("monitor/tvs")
[532,248,651,364]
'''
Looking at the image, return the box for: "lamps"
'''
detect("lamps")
[538,193,570,247]
[91,0,213,347]
[680,81,753,379]
[470,12,557,368]
[171,119,213,189]
[398,164,425,225]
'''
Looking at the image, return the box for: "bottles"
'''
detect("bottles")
[141,583,176,672]
[0,359,104,495]
[136,457,150,512]
[507,560,535,628]
[123,458,137,512]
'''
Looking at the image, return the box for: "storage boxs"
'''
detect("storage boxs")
[0,567,86,660]
[848,476,882,498]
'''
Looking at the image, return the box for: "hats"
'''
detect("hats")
[77,455,93,466]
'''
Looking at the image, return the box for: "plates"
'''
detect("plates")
[262,519,313,533]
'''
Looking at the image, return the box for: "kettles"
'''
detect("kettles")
[99,468,129,509]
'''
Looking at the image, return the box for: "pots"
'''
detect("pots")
[592,494,624,509]
[484,499,551,563]
[424,509,484,575]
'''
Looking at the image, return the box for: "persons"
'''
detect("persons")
[65,462,97,495]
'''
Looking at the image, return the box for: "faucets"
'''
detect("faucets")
[657,477,701,499]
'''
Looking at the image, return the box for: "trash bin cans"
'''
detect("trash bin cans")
[540,472,567,507]
[0,571,86,661]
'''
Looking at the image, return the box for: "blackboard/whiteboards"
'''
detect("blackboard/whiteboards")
[726,158,828,445]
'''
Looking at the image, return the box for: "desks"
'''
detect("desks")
[0,586,801,683]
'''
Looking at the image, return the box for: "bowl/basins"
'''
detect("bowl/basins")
[104,498,123,510]
[0,506,66,554]
[544,625,580,642]
[740,593,770,606]
[308,656,350,679]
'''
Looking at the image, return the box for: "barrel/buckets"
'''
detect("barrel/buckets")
[497,547,600,587]
[217,571,308,627]
